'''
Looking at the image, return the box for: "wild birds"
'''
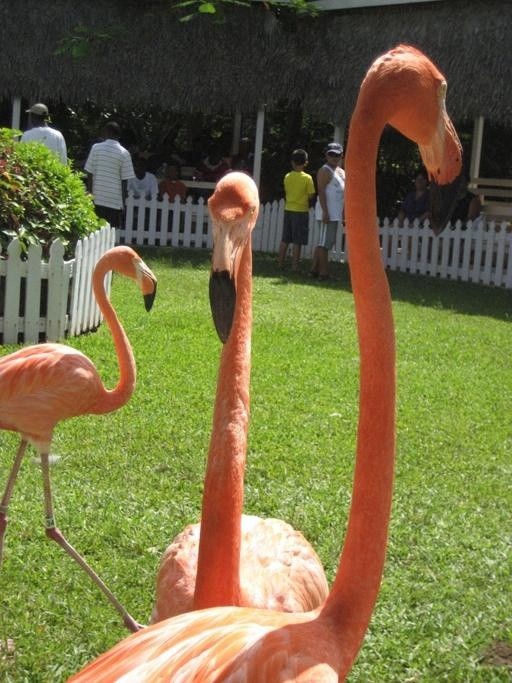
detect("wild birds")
[56,33,463,682]
[1,242,159,638]
[150,167,334,618]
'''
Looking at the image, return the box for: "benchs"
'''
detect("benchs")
[150,177,216,223]
[178,166,197,179]
[464,178,512,248]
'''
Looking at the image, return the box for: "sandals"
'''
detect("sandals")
[307,270,328,281]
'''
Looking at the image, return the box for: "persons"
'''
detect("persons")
[429,170,482,245]
[386,170,437,257]
[277,148,317,272]
[125,153,160,201]
[82,116,136,230]
[17,101,68,169]
[158,165,197,205]
[377,148,399,204]
[307,141,346,277]
[144,143,188,170]
[193,143,295,205]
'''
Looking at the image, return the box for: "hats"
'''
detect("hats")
[25,103,49,117]
[324,142,343,155]
